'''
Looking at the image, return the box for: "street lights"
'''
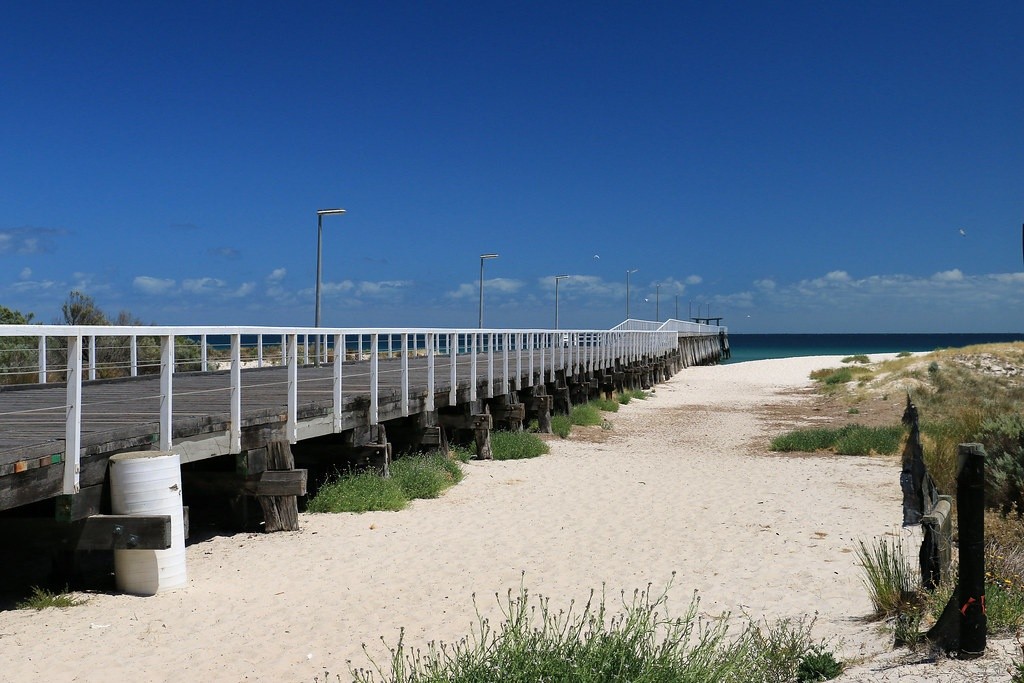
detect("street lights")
[554,275,569,329]
[314,208,346,366]
[625,268,640,318]
[657,282,663,322]
[477,253,499,329]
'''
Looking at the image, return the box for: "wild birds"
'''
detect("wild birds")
[592,254,600,259]
[747,314,750,318]
[960,228,966,236]
[642,298,648,303]
